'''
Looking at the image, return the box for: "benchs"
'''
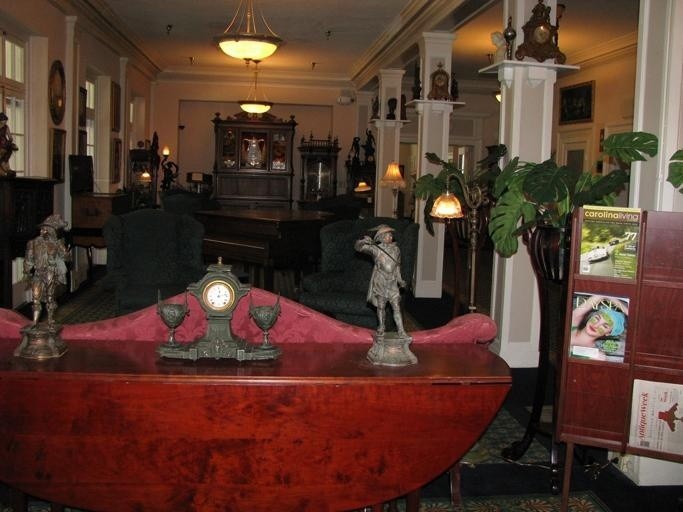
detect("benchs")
[2,286,496,511]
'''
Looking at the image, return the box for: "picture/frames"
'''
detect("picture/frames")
[47,59,66,125]
[111,137,120,184]
[79,86,87,127]
[558,80,595,125]
[110,80,120,132]
[50,128,65,184]
[78,130,87,156]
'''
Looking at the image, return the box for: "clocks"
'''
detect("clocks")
[156,255,284,361]
[514,0,566,64]
[428,61,452,101]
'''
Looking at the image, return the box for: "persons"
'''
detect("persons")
[355,225,409,339]
[349,137,361,161]
[365,127,376,161]
[0,112,17,177]
[22,214,68,332]
[570,294,629,362]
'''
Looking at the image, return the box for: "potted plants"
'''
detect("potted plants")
[485,134,658,281]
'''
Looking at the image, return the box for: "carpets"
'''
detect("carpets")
[361,489,611,512]
[40,272,559,472]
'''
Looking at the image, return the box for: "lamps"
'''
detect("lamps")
[428,173,490,313]
[379,160,407,218]
[238,72,273,117]
[213,0,284,71]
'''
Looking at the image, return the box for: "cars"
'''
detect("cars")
[587,246,609,263]
[608,239,620,247]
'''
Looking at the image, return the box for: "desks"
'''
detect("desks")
[0,338,513,512]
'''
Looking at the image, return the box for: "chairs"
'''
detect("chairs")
[101,207,208,317]
[300,216,419,331]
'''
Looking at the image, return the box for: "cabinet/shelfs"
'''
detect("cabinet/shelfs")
[0,174,54,322]
[553,203,683,512]
